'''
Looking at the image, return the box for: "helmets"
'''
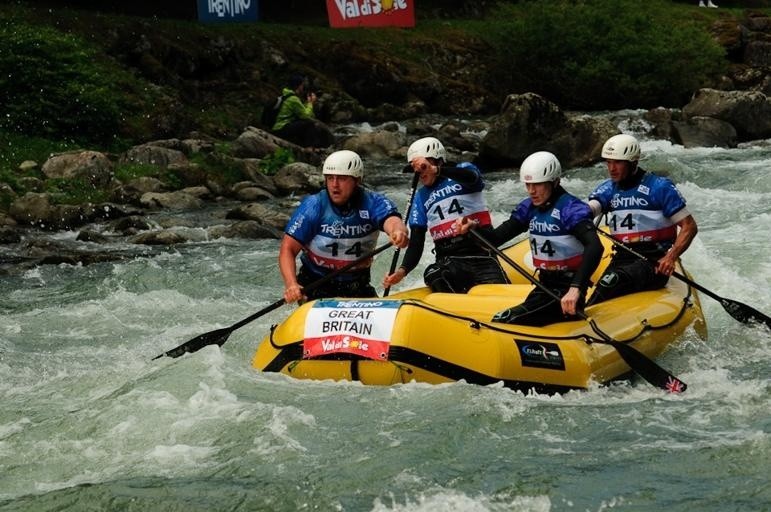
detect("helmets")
[322,150,364,182]
[601,133,641,163]
[407,136,447,165]
[519,151,562,183]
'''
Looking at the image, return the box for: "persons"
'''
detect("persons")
[586,134,698,305]
[269,73,317,148]
[383,136,511,294]
[279,150,409,306]
[455,151,604,327]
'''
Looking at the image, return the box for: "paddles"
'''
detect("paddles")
[383,164,427,294]
[461,217,687,394]
[590,223,770,333]
[150,236,410,359]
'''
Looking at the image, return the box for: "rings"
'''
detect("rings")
[666,270,669,273]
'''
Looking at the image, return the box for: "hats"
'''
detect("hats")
[288,74,305,91]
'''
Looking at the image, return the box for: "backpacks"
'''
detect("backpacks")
[261,91,283,125]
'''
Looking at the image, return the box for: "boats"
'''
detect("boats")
[251,224,707,398]
